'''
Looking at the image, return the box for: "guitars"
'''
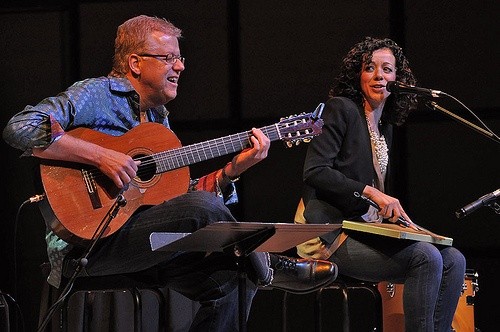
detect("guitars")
[33,103,325,248]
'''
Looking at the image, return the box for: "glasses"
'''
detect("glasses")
[138,54,185,65]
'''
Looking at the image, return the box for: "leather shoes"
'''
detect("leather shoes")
[258,254,338,294]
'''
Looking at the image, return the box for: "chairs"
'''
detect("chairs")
[282,254,380,332]
[41,218,165,332]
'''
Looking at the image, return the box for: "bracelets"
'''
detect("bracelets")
[222,162,242,183]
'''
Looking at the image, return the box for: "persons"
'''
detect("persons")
[4,14,337,331]
[299,37,467,332]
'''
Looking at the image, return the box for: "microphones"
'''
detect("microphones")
[386,80,443,96]
[79,257,88,273]
